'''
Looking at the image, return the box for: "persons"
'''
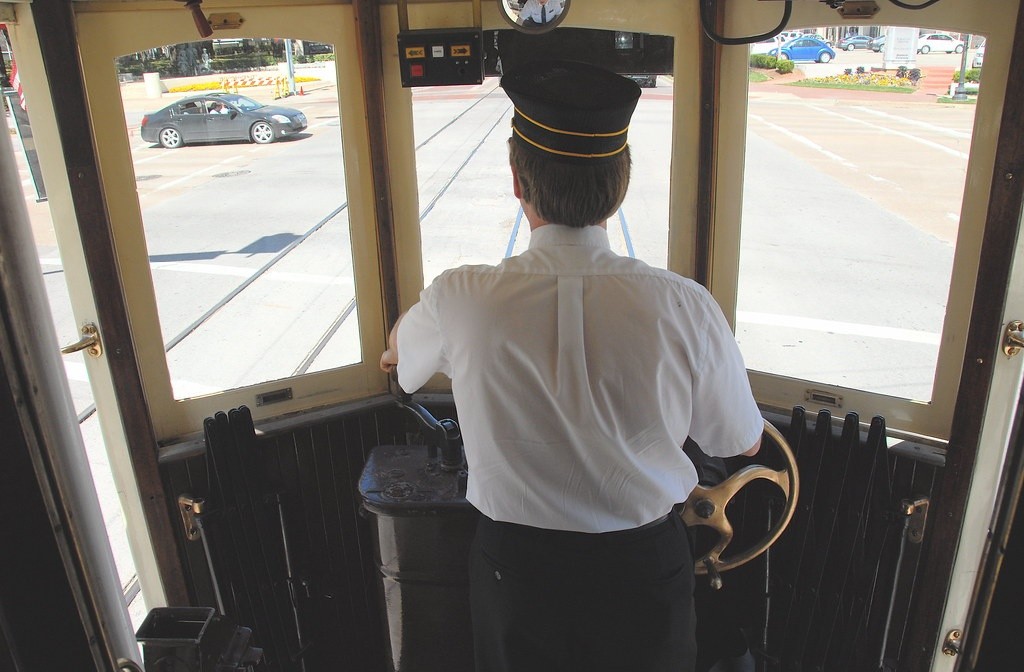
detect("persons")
[380,59,765,672]
[220,102,235,114]
[209,102,222,114]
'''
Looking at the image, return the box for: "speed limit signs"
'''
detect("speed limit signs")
[274,77,281,100]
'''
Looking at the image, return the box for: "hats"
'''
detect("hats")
[501,61,641,159]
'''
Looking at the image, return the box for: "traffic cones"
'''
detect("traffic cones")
[282,77,290,98]
[299,86,305,96]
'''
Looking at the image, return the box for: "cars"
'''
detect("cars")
[835,36,873,51]
[140,92,308,148]
[749,38,778,54]
[916,33,964,54]
[972,39,987,68]
[799,34,832,48]
[774,35,788,45]
[867,35,886,52]
[767,37,836,63]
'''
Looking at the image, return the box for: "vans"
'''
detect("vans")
[781,31,799,40]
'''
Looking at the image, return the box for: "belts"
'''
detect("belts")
[601,513,674,537]
